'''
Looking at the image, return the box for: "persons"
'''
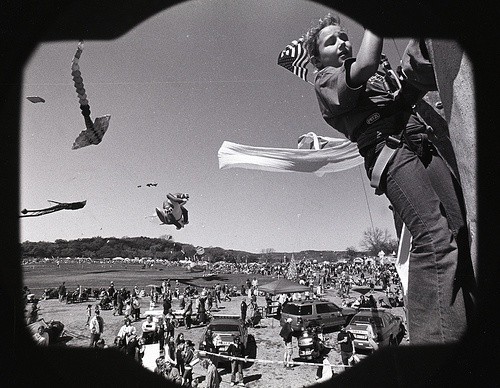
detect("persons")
[32,326,49,346]
[88,310,104,348]
[114,336,125,355]
[141,279,165,350]
[199,330,220,367]
[296,255,404,305]
[135,338,145,364]
[117,318,137,338]
[189,351,212,386]
[174,333,185,377]
[296,318,357,384]
[307,14,474,346]
[22,279,141,326]
[279,318,294,367]
[227,336,248,386]
[22,255,208,270]
[164,336,177,366]
[127,334,138,359]
[181,340,195,386]
[165,278,296,336]
[208,257,296,278]
[154,358,165,376]
[164,361,181,384]
[198,359,219,388]
[96,339,105,348]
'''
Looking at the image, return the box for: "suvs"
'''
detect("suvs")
[280,301,357,334]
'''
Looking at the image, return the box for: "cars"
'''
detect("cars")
[344,311,405,355]
[199,319,256,368]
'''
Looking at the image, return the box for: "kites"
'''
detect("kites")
[71,39,111,150]
[27,96,45,103]
[155,193,189,230]
[20,200,87,218]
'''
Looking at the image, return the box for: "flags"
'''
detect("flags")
[277,14,342,81]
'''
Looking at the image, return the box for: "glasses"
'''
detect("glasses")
[235,339,239,341]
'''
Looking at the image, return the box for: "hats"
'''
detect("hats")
[287,317,293,322]
